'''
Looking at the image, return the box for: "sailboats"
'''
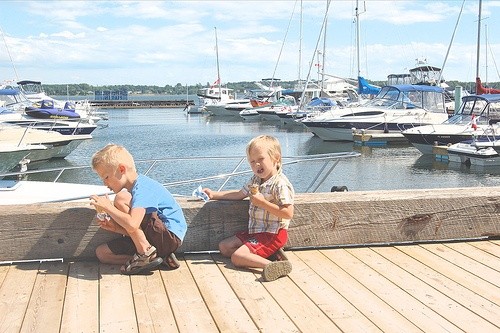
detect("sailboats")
[182,0,500,166]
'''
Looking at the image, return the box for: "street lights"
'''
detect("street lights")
[213,26,221,96]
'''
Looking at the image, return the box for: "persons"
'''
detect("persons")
[268,105,294,113]
[90,144,187,276]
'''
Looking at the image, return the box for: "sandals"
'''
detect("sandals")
[120,245,163,274]
[157,252,180,270]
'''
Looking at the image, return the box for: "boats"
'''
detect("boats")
[0,150,364,206]
[1,28,110,181]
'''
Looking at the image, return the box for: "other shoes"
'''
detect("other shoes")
[262,259,292,282]
[272,247,289,261]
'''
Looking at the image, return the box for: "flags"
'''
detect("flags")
[202,135,294,281]
[214,79,220,85]
